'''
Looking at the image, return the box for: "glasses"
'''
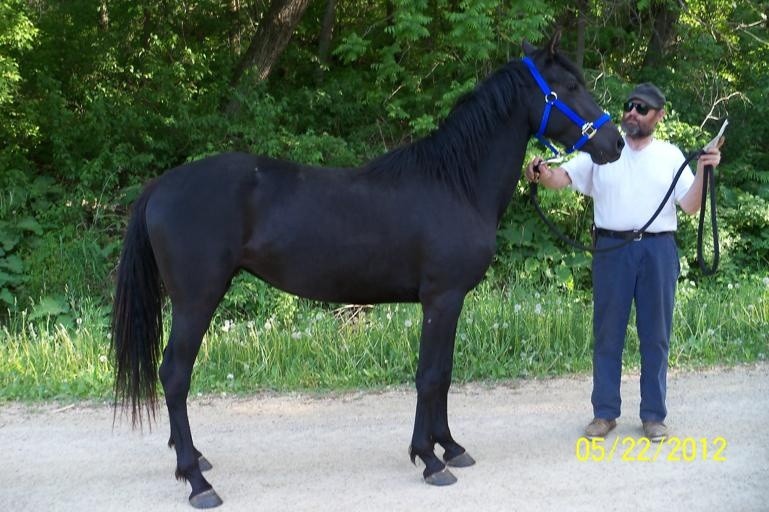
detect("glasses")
[623,102,648,114]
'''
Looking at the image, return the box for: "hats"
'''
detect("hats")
[627,82,665,109]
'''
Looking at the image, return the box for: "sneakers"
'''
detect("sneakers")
[642,421,668,442]
[586,418,616,440]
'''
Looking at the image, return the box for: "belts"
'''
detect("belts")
[597,229,668,240]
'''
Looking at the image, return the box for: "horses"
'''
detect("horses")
[107,26,626,509]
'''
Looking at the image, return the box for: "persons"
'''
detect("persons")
[524,82,725,439]
